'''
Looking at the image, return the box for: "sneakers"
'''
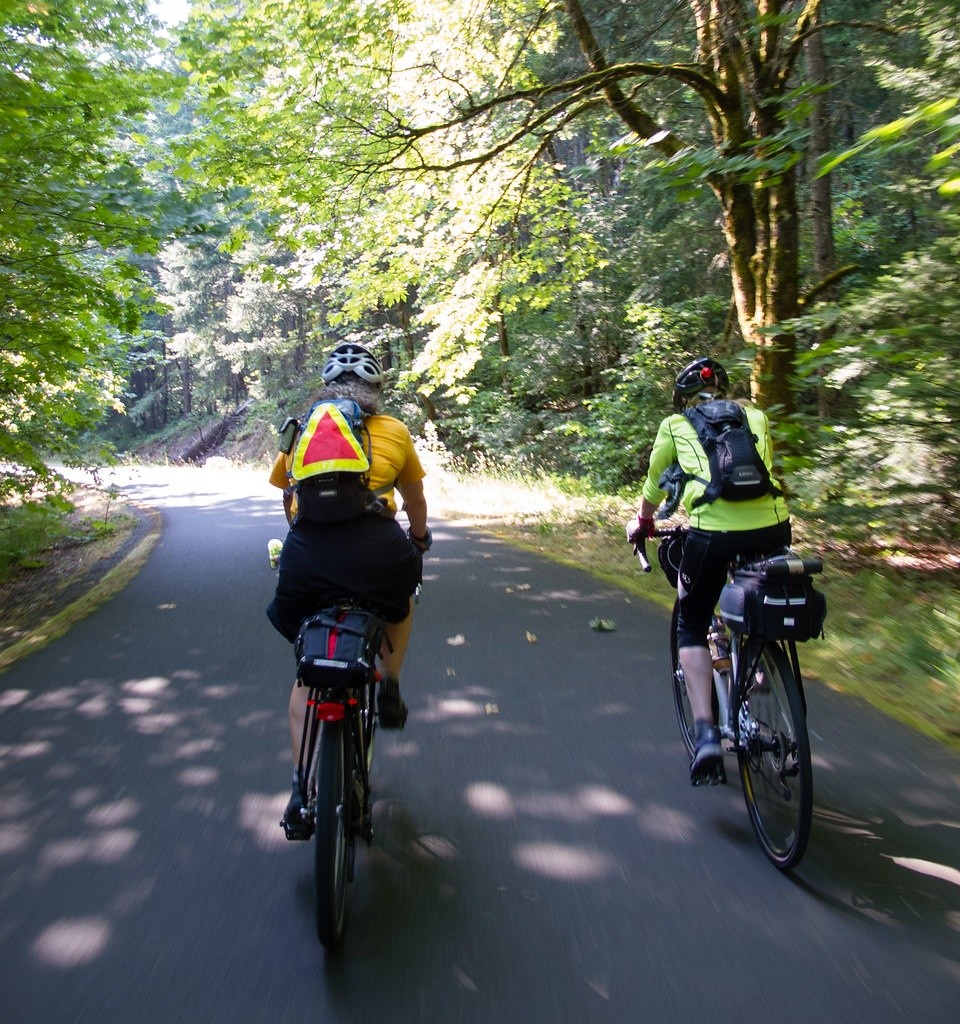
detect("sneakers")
[284,784,314,843]
[689,723,723,789]
[377,676,408,732]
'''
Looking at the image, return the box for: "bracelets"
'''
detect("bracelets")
[637,513,654,541]
[409,526,430,541]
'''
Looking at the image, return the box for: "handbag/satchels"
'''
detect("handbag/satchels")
[717,564,828,644]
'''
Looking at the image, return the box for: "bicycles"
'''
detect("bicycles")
[279,538,428,951]
[629,526,828,870]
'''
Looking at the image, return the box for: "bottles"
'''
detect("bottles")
[708,615,731,672]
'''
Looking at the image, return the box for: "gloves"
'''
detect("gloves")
[625,516,654,546]
[406,529,433,555]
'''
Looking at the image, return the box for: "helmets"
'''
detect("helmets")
[322,345,385,388]
[672,358,729,407]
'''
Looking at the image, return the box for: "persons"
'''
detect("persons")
[267,343,431,841]
[626,360,792,786]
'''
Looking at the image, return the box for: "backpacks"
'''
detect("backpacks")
[681,401,787,509]
[296,604,393,692]
[276,397,398,543]
